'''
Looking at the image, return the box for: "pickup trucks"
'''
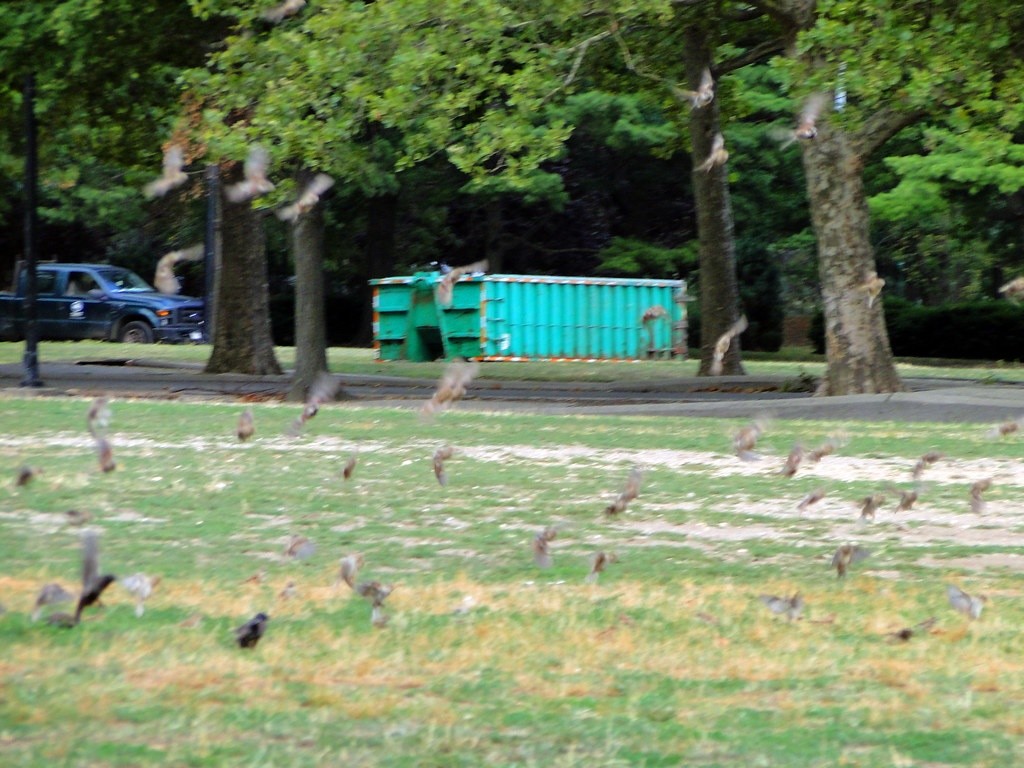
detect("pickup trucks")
[0,259,208,345]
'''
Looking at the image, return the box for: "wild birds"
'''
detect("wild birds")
[1,0,1024,649]
